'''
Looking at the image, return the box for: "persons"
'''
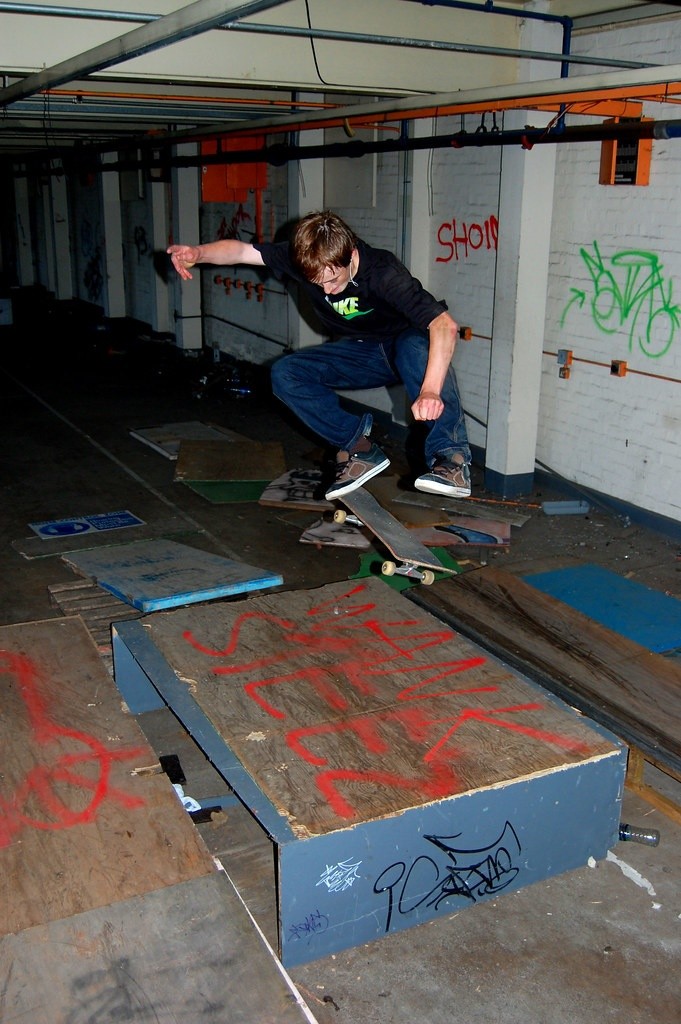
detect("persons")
[167,210,472,502]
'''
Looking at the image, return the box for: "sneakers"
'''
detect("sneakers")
[325,443,390,501]
[414,450,471,499]
